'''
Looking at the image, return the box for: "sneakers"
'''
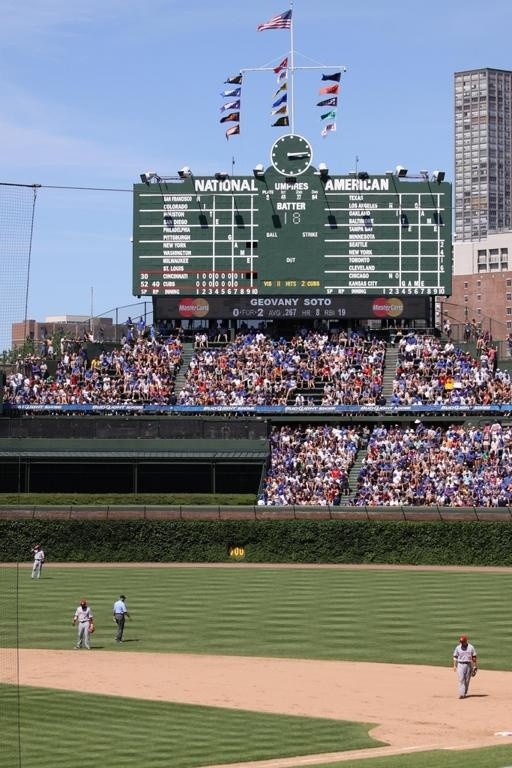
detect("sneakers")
[458,694,467,699]
[73,637,124,650]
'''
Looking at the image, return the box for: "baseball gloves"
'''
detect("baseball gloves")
[472,667,477,676]
[89,624,95,633]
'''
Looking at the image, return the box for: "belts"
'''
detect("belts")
[458,662,471,664]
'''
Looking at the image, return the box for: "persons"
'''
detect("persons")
[1,317,185,417]
[391,319,511,416]
[180,321,387,416]
[112,593,132,643]
[71,599,95,649]
[251,416,512,510]
[453,633,478,699]
[30,544,45,579]
[256,419,512,506]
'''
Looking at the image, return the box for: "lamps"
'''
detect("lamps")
[135,164,268,186]
[316,161,449,186]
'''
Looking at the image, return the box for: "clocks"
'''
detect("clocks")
[269,133,313,175]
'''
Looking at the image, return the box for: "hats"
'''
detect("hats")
[80,594,126,607]
[459,635,468,643]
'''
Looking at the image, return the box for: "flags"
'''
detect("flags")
[253,8,293,31]
[219,57,343,141]
